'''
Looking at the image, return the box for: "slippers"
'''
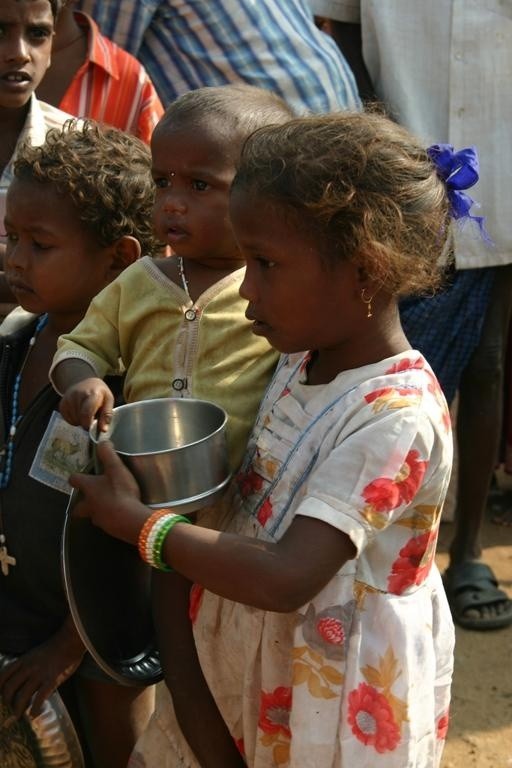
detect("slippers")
[441,559,511,631]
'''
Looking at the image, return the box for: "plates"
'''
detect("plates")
[0,651,89,768]
[56,451,197,691]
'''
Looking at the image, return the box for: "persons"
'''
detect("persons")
[2,0,512,767]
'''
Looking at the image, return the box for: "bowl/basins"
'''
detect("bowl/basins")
[89,395,232,519]
